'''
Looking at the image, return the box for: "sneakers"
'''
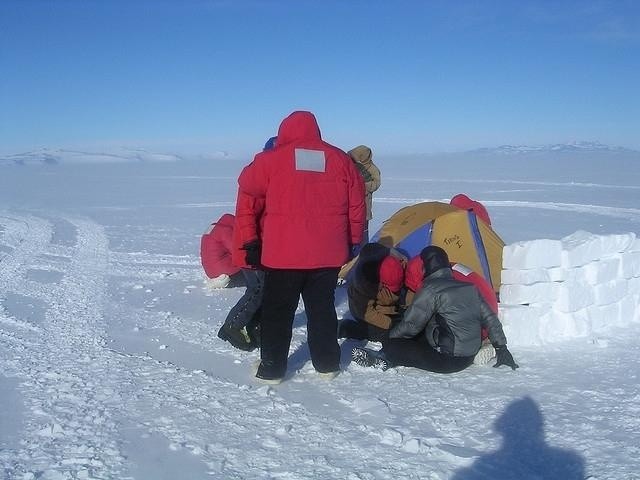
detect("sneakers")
[474,338,496,365]
[217,323,256,351]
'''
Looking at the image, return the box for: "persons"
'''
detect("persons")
[240,109,364,385]
[200,213,266,351]
[347,145,380,242]
[337,243,518,371]
[451,194,490,225]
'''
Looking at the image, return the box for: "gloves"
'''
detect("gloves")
[243,240,260,265]
[493,345,519,370]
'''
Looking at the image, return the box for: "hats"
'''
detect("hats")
[404,255,426,292]
[377,255,404,293]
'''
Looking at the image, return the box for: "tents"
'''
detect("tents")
[337,201,506,303]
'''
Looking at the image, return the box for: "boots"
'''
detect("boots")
[351,347,396,371]
